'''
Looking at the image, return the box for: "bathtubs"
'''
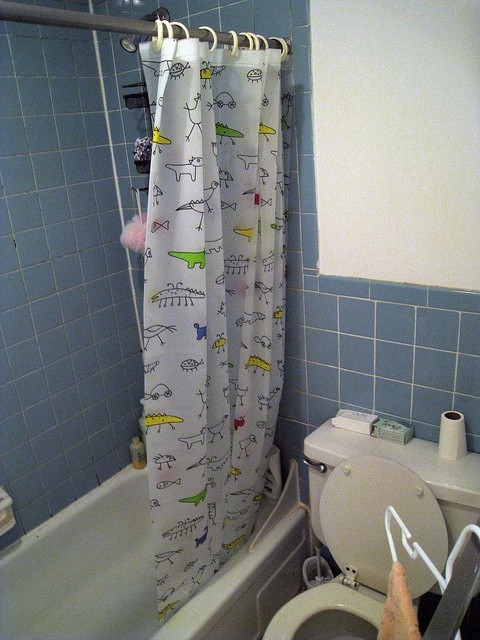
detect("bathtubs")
[0,454,306,640]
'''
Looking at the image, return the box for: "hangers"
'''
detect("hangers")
[384,503,479,635]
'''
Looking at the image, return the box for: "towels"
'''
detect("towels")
[376,562,423,640]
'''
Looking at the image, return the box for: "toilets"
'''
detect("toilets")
[260,416,480,640]
